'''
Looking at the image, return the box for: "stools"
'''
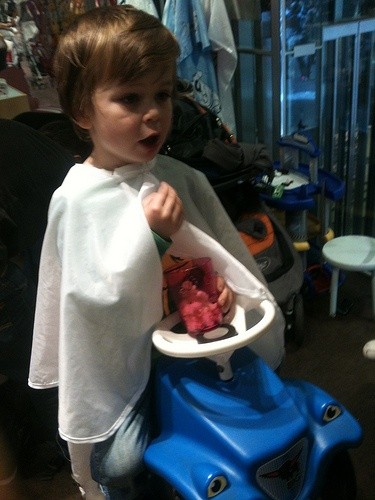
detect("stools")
[322,235,375,320]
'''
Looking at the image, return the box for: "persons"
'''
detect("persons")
[0,1,124,90]
[27,6,286,500]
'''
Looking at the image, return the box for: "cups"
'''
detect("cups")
[164,256,223,336]
